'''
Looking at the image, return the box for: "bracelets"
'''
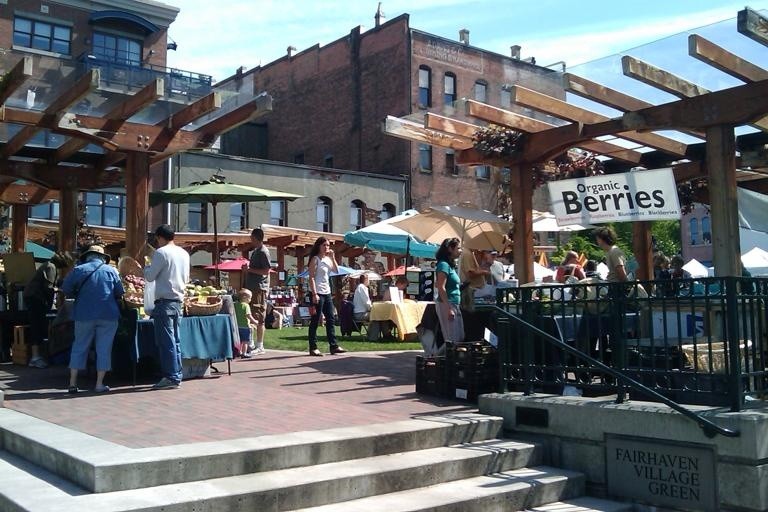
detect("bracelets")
[312,293,317,296]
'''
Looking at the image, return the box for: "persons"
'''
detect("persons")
[353,275,381,342]
[308,237,348,357]
[593,226,632,379]
[457,247,756,341]
[24,250,72,369]
[235,287,259,359]
[241,228,271,353]
[0,272,15,364]
[59,245,124,394]
[433,236,465,356]
[142,224,190,390]
[382,276,410,339]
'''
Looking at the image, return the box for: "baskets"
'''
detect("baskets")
[123,291,144,309]
[187,295,224,316]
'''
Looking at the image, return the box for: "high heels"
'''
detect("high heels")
[330,347,349,355]
[310,349,324,356]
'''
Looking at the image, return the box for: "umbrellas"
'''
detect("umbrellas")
[384,265,409,276]
[298,262,360,278]
[204,256,278,290]
[345,268,383,281]
[0,240,59,263]
[493,209,598,233]
[148,172,310,289]
[344,209,450,282]
[388,201,516,284]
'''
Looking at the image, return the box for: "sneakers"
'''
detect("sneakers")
[95,385,110,395]
[152,372,183,391]
[236,346,266,359]
[28,357,48,369]
[383,335,397,342]
[69,384,79,395]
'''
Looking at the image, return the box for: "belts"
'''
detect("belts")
[154,298,183,305]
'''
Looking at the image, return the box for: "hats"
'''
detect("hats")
[490,250,497,255]
[470,270,489,279]
[79,245,111,264]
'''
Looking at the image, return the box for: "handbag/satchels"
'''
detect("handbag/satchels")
[45,288,55,314]
[565,264,579,285]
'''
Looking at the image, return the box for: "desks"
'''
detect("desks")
[111,293,241,387]
[515,309,641,374]
[267,301,436,344]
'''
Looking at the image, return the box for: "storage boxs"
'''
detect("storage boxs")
[415,338,500,406]
